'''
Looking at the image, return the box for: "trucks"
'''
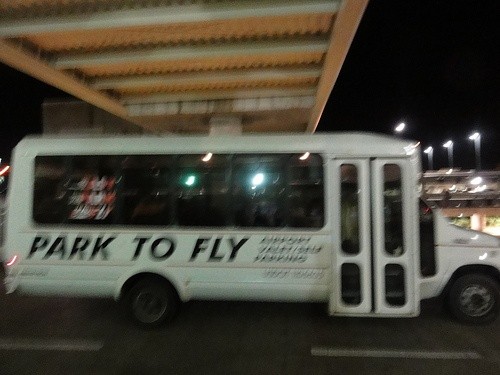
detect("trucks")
[1,133,499,329]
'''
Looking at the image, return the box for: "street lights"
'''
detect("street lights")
[442,140,454,171]
[424,146,433,170]
[469,132,480,171]
